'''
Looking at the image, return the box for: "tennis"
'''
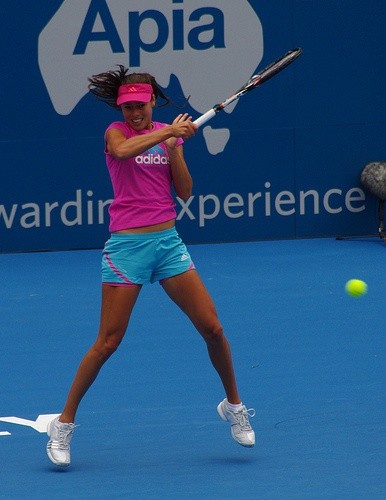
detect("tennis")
[345,278,368,296]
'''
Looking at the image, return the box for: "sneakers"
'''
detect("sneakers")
[47,415,80,466]
[216,397,257,447]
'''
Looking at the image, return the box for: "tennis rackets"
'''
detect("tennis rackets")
[192,48,303,128]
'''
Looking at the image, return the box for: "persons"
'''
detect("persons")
[47,64,255,466]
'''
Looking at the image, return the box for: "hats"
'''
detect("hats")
[116,83,153,105]
